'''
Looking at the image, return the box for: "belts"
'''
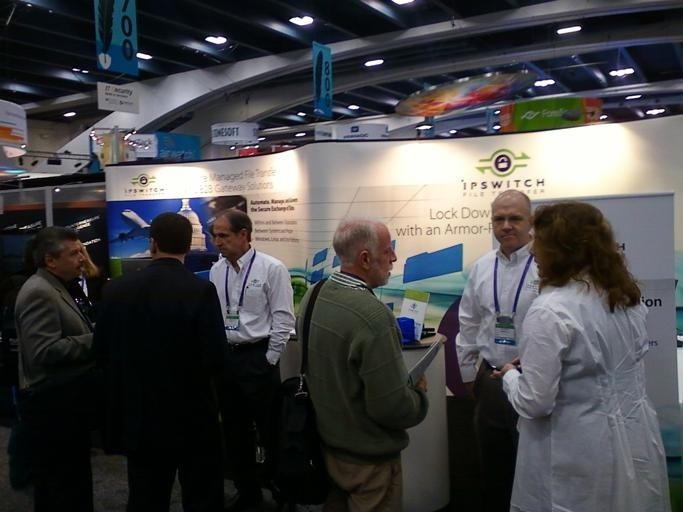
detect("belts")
[229,344,243,353]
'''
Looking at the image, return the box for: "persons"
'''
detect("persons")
[87,153,100,174]
[23,235,109,317]
[14,225,94,512]
[297,218,429,512]
[87,212,230,512]
[454,189,541,512]
[209,209,297,512]
[487,202,671,512]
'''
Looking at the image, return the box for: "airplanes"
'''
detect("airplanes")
[108,208,153,245]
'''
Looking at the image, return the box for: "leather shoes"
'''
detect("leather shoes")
[272,503,297,512]
[223,488,264,511]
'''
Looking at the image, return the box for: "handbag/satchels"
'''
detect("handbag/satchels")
[252,378,328,506]
